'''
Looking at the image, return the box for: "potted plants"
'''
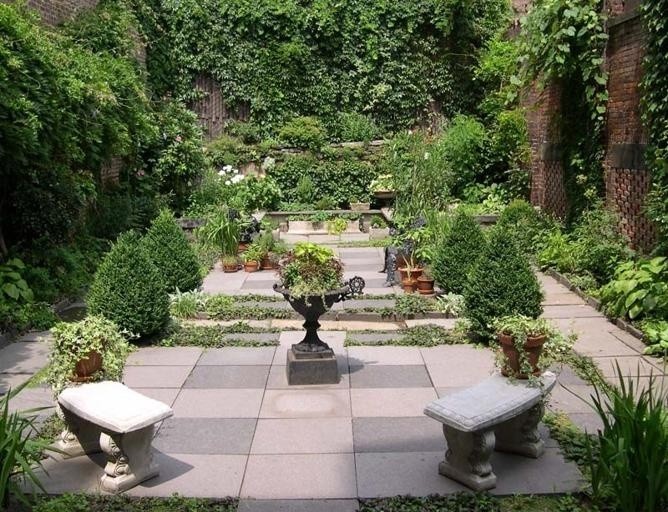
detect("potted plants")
[26,314,142,406]
[485,314,601,402]
[200,205,285,273]
[398,249,438,295]
[269,242,351,354]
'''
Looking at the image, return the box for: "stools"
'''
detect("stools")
[424,367,556,492]
[58,378,173,494]
